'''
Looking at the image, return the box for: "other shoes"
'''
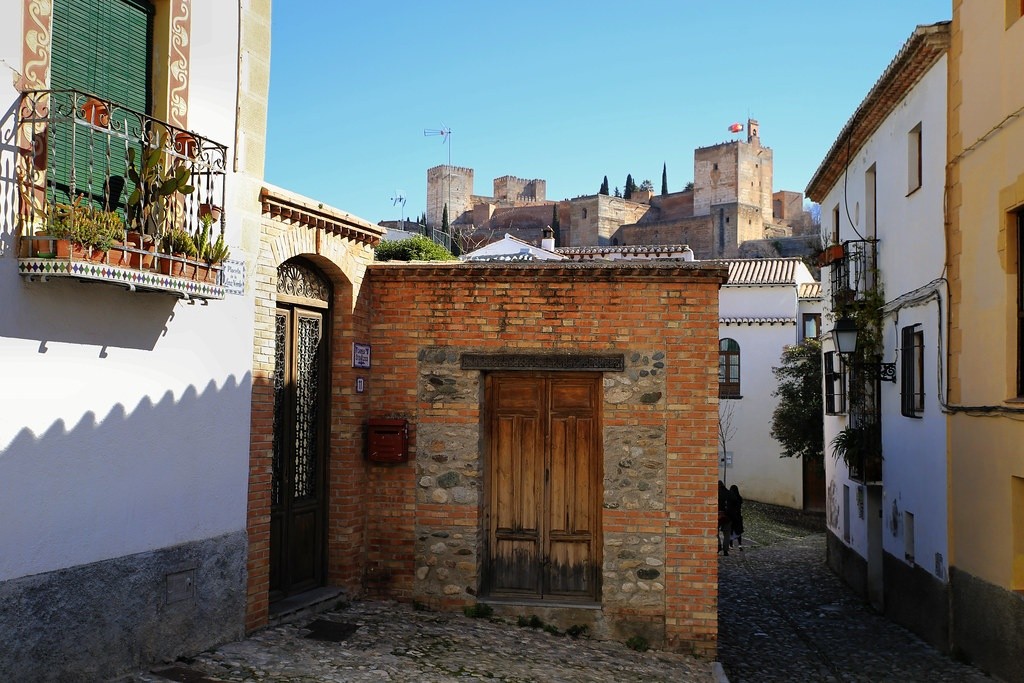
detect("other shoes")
[738,545,743,551]
[730,546,734,550]
[724,551,728,556]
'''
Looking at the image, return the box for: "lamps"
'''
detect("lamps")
[828,304,896,384]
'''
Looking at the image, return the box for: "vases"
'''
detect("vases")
[81,99,108,128]
[200,204,221,222]
[175,132,197,159]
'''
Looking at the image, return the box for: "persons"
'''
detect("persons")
[718,479,744,556]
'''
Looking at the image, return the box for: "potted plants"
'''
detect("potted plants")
[833,426,885,469]
[22,129,231,284]
